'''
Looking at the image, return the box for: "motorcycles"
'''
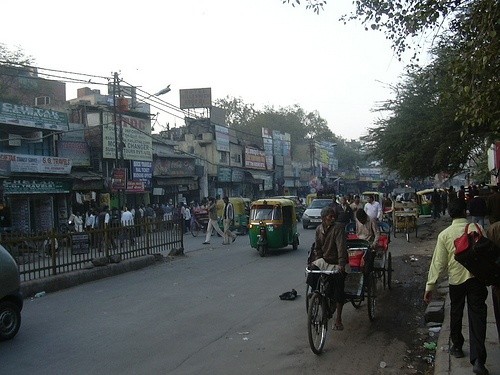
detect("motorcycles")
[248,194,318,257]
[415,188,446,216]
[214,197,253,234]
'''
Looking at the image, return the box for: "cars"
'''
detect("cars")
[301,199,335,229]
[0,244,24,341]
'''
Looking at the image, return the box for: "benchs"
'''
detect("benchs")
[348,234,388,250]
[347,247,369,273]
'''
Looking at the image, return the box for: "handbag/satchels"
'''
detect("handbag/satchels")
[454,222,495,286]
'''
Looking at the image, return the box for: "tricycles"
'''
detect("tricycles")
[304,191,418,355]
[190,212,211,237]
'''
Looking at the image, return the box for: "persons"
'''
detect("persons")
[355,207,380,268]
[424,198,500,375]
[430,183,500,229]
[202,198,225,245]
[315,207,348,330]
[328,193,403,234]
[67,197,208,252]
[221,196,237,245]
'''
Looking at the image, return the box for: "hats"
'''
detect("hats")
[222,197,229,201]
[101,204,109,210]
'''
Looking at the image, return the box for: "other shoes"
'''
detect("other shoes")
[130,241,134,246]
[121,243,125,248]
[472,359,490,375]
[446,346,464,358]
[112,245,118,249]
[334,322,343,331]
[231,237,235,242]
[223,241,230,245]
[95,248,102,252]
[203,242,211,245]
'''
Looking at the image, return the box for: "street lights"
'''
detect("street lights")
[310,143,338,184]
[114,84,171,224]
